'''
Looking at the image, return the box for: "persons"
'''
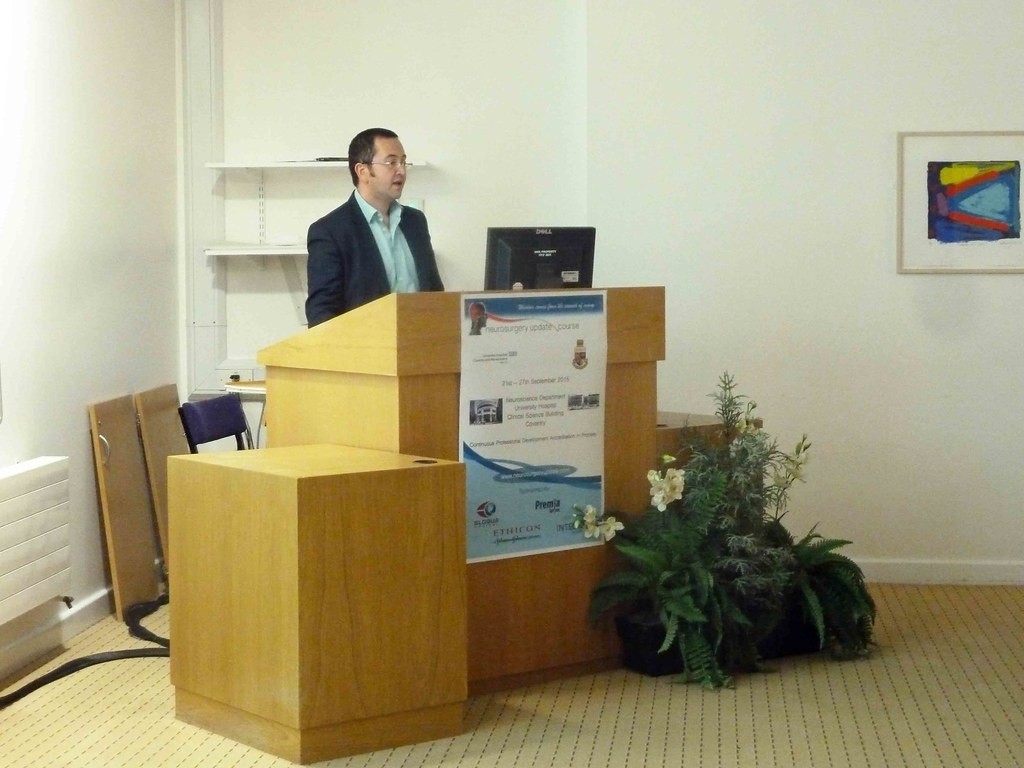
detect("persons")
[305,128,444,330]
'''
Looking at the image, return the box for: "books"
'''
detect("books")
[225,381,267,394]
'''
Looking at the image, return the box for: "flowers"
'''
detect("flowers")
[570,372,879,691]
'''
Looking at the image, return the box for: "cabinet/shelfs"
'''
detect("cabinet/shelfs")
[209,163,421,259]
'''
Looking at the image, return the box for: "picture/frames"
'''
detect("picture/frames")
[894,131,1024,273]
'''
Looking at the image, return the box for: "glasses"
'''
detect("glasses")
[360,159,414,172]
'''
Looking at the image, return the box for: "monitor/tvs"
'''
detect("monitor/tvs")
[482,227,596,291]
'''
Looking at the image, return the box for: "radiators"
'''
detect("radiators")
[0,455,72,626]
